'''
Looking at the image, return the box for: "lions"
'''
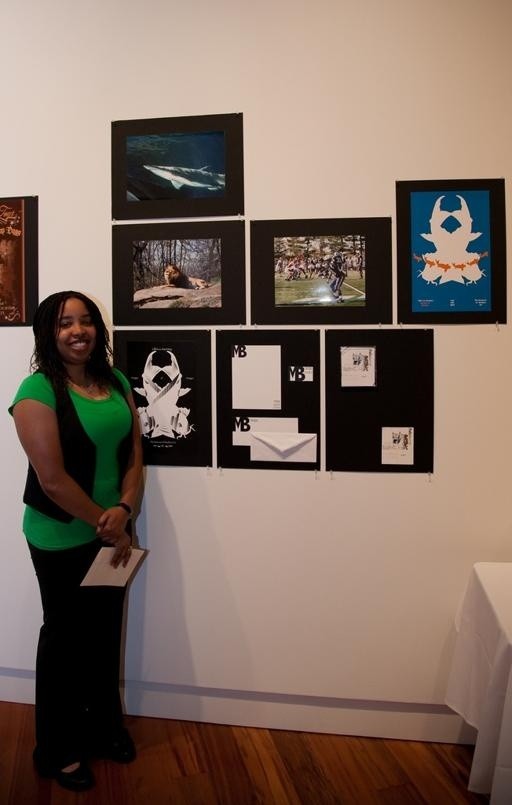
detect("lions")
[164,264,212,290]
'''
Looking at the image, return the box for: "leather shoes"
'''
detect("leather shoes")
[32,752,94,790]
[102,733,135,763]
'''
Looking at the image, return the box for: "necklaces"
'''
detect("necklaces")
[68,376,101,397]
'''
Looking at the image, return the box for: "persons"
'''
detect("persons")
[7,290,150,796]
[276,245,366,307]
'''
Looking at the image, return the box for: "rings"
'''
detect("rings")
[127,545,133,551]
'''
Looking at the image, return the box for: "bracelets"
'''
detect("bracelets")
[115,502,132,517]
